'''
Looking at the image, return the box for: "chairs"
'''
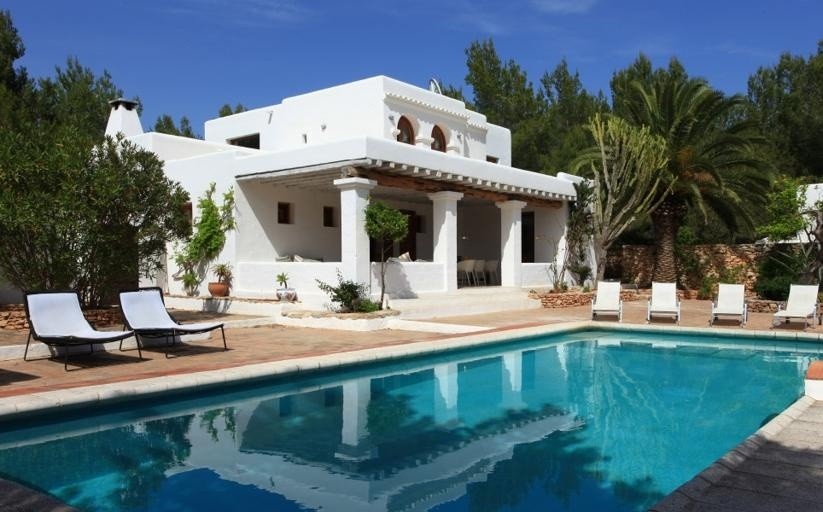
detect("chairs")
[457,259,500,286]
[590,280,819,331]
[24,287,226,370]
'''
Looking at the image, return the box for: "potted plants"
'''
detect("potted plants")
[314,266,370,312]
[276,272,295,300]
[208,261,232,296]
